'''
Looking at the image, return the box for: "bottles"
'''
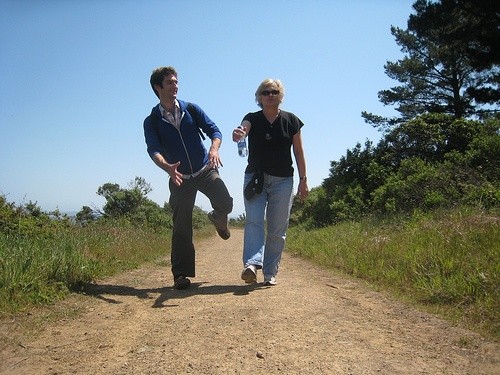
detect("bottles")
[236,126,248,157]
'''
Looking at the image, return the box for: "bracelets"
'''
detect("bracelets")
[300,176,308,180]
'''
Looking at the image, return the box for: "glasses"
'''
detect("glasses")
[258,89,280,98]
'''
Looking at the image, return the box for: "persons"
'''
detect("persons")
[142,66,234,298]
[233,78,308,287]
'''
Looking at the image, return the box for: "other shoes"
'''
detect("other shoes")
[174,275,191,289]
[207,211,231,240]
[241,264,258,285]
[264,274,276,286]
